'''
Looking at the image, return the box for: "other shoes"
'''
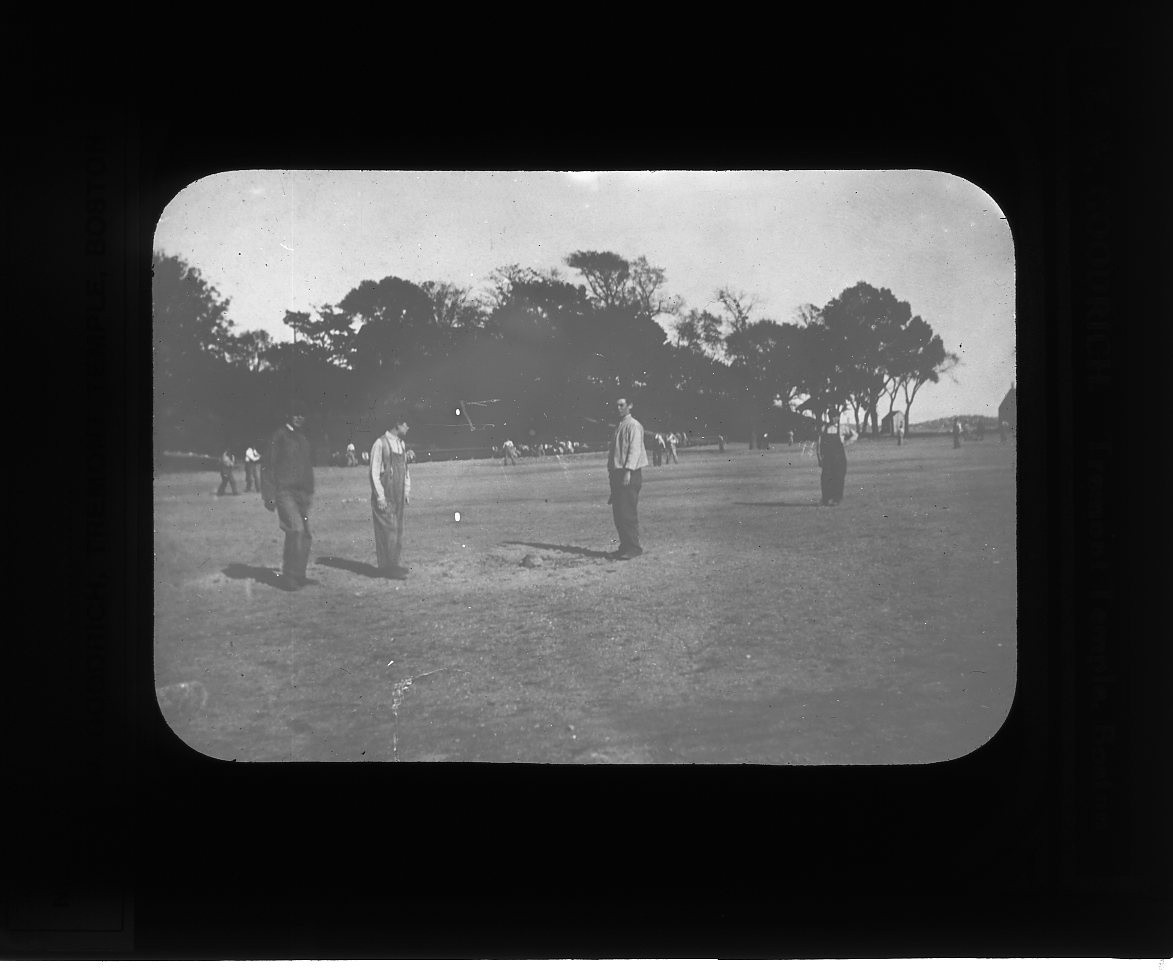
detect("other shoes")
[379,565,409,579]
[296,577,319,585]
[283,574,298,590]
[605,548,642,560]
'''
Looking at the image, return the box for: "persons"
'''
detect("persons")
[653,417,1009,467]
[216,438,575,497]
[369,411,412,578]
[817,408,858,507]
[606,396,648,560]
[261,409,318,591]
